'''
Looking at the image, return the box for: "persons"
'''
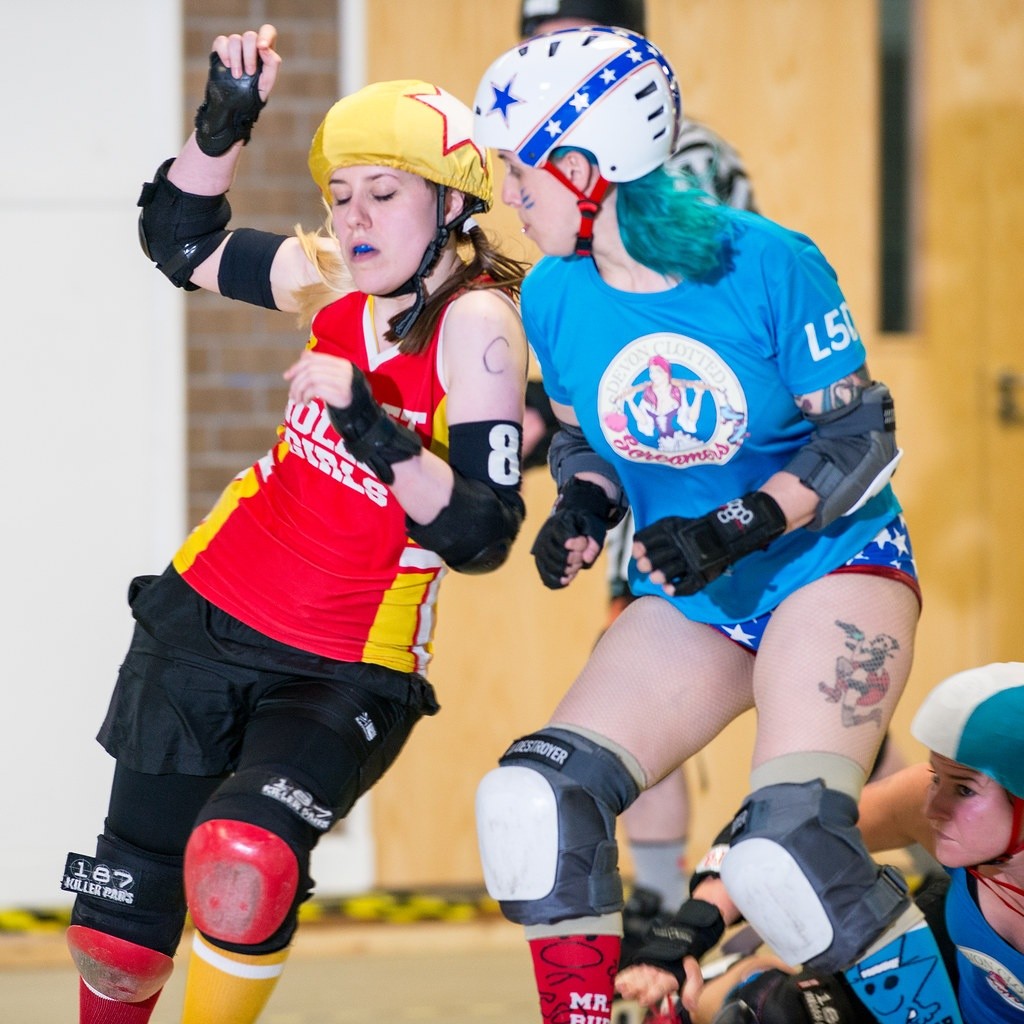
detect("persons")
[60,23,529,1024]
[475,24,966,1024]
[603,117,891,918]
[611,661,1024,1024]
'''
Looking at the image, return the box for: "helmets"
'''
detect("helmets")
[471,26,678,182]
[910,661,1023,798]
[305,78,491,219]
[514,0,645,44]
